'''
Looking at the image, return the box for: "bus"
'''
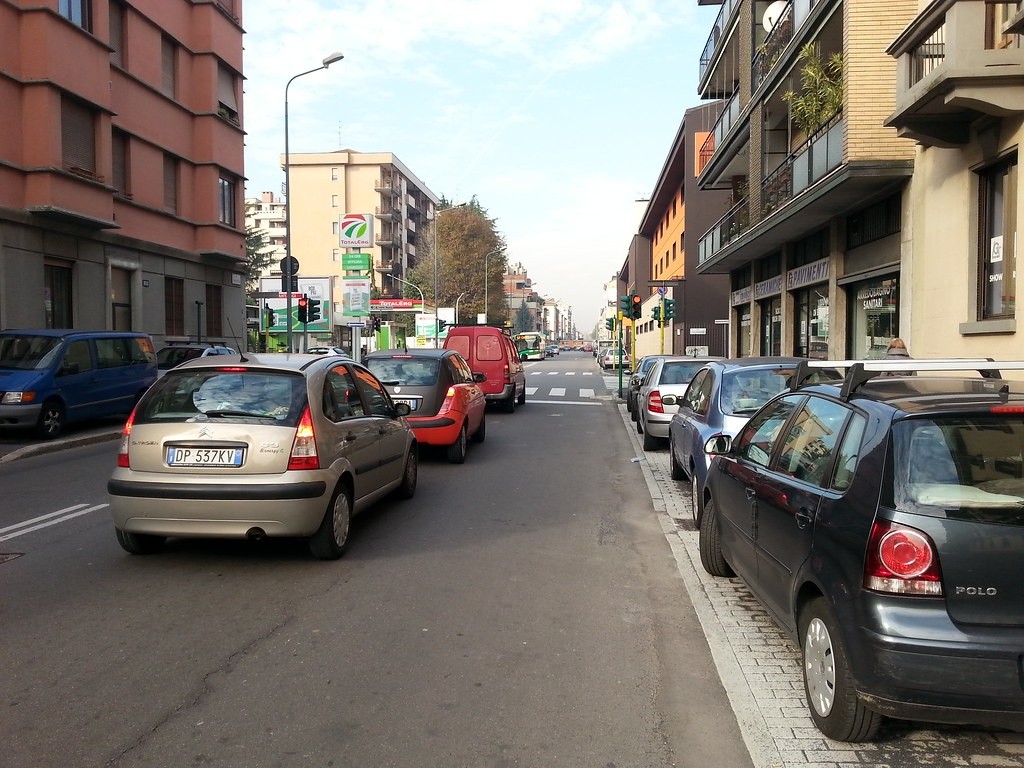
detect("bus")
[513,332,545,361]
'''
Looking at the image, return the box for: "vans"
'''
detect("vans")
[441,326,525,414]
[0,328,157,440]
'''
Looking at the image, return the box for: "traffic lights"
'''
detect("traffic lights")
[308,299,320,321]
[631,294,641,320]
[439,320,446,332]
[665,299,677,321]
[651,306,659,320]
[620,296,631,318]
[606,318,613,331]
[298,299,307,321]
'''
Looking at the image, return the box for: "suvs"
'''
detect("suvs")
[698,357,1023,744]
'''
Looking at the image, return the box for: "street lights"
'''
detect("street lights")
[485,246,508,324]
[387,273,424,349]
[433,202,467,349]
[284,52,346,353]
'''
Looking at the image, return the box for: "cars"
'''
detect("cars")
[635,356,727,452]
[545,344,630,370]
[360,349,487,463]
[623,355,659,413]
[156,347,236,381]
[668,355,845,530]
[107,353,419,562]
